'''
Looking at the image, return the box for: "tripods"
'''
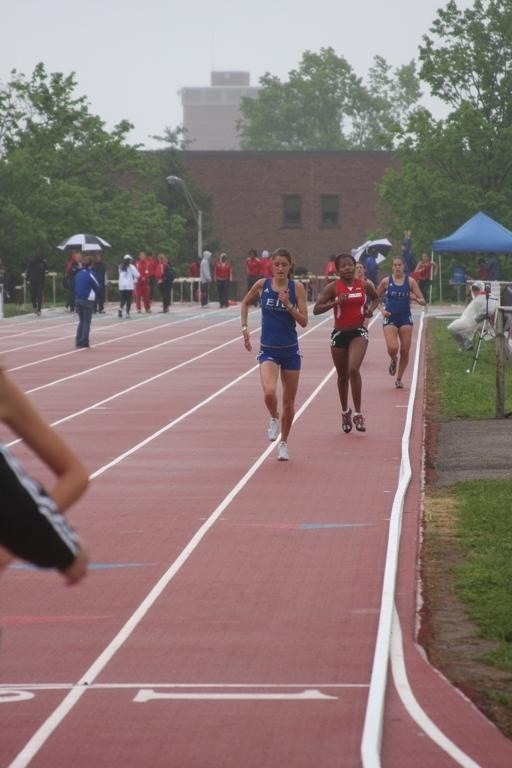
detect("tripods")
[471,293,509,374]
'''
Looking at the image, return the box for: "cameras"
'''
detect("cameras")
[485,283,491,293]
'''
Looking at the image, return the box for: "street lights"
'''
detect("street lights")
[164,173,204,259]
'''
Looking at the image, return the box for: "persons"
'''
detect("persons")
[326,254,378,337]
[313,254,379,433]
[25,254,50,316]
[65,250,105,348]
[190,250,237,308]
[447,282,495,351]
[376,255,427,388]
[401,231,437,303]
[245,248,271,310]
[117,251,174,318]
[452,254,498,292]
[0,363,90,584]
[0,443,88,587]
[241,248,308,460]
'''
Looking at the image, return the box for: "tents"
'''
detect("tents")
[429,211,512,309]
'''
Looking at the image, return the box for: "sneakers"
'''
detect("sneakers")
[353,414,366,431]
[390,359,397,375]
[396,379,403,387]
[341,406,352,432]
[268,417,280,440]
[278,440,288,460]
